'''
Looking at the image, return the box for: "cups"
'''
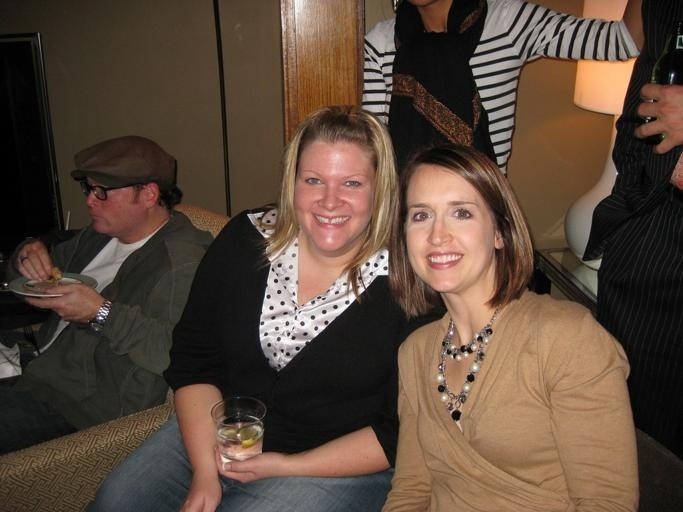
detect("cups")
[211,395,267,473]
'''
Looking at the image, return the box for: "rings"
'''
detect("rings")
[21,257,27,264]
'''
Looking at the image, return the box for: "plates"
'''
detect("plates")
[11,273,98,298]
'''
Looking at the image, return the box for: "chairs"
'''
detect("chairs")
[1,205,232,511]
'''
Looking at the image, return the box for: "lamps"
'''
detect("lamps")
[565,0,646,273]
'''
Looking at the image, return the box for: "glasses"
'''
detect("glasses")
[77,180,141,200]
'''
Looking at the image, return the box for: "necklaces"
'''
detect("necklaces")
[434,309,500,422]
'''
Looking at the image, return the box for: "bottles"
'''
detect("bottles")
[637,20,683,143]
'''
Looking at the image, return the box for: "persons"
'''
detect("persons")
[0,136,215,458]
[355,0,642,192]
[553,0,683,458]
[84,105,443,512]
[380,142,644,512]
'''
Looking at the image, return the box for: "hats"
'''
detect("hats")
[70,137,176,187]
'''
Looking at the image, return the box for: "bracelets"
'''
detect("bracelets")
[87,297,111,331]
